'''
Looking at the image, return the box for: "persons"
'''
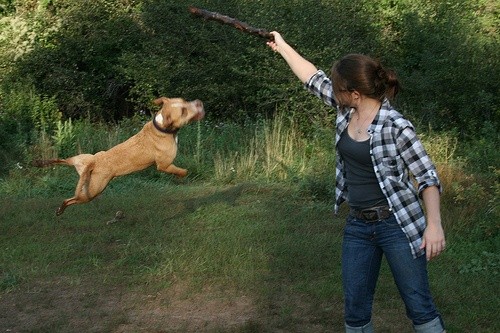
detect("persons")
[266,32,446,333]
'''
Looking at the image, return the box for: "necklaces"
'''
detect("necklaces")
[357,100,378,133]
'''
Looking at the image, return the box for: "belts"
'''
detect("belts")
[349,207,394,222]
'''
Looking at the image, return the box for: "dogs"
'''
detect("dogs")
[26,96,205,218]
